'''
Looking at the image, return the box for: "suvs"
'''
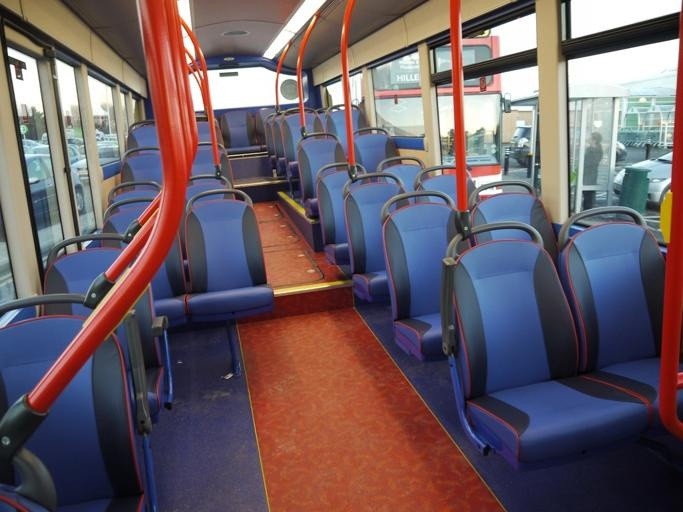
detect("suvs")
[507,125,531,168]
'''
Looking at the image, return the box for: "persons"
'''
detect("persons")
[582,131,603,211]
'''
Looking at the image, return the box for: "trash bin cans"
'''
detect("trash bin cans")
[618,168,651,221]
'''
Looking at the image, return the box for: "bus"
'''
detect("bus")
[348,32,504,203]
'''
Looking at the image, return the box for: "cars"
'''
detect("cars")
[612,150,671,207]
[17,128,119,182]
[21,154,85,218]
[567,125,627,165]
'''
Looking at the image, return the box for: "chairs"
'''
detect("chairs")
[431,198,682,511]
[220,108,260,155]
[41,238,176,415]
[103,107,278,380]
[255,104,479,307]
[1,293,162,511]
[379,180,560,365]
